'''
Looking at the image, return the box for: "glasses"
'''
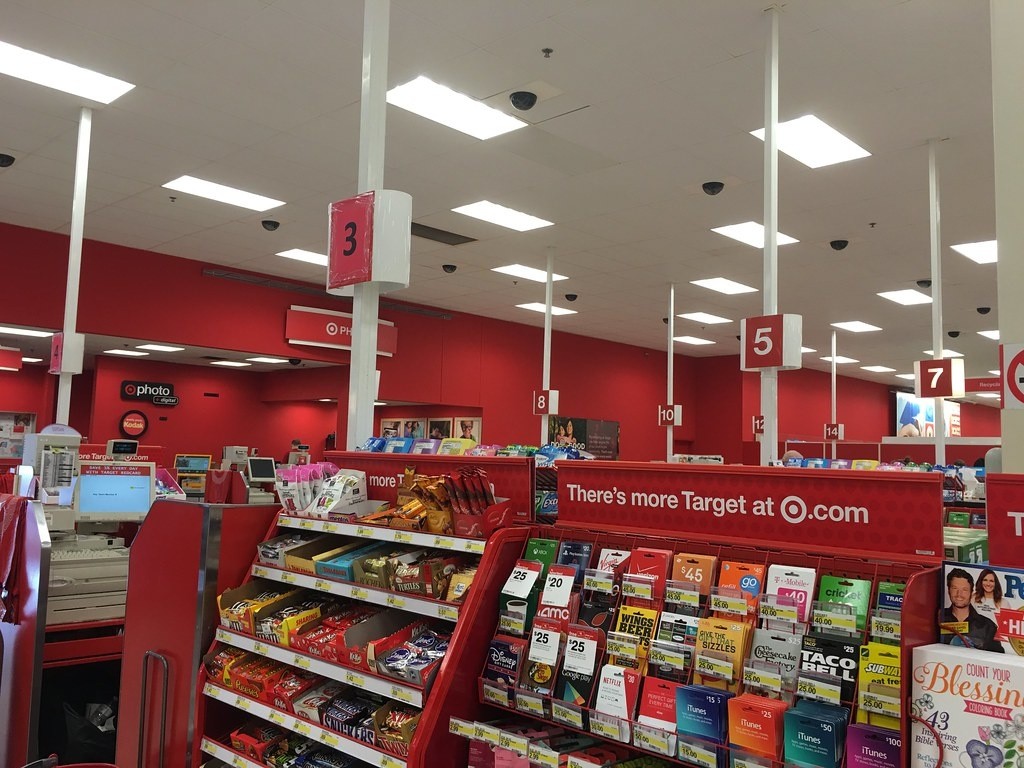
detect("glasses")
[461,424,472,431]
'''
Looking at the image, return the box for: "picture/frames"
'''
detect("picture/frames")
[427,418,453,439]
[403,418,427,439]
[380,418,403,438]
[453,417,482,446]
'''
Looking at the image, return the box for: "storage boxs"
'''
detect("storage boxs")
[217,579,448,685]
[911,643,1024,768]
[944,512,989,566]
[285,469,512,542]
[204,644,423,768]
[787,457,986,504]
[362,436,479,457]
[257,534,472,603]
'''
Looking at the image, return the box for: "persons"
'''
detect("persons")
[557,424,575,445]
[384,421,401,439]
[429,424,449,440]
[17,417,29,428]
[411,421,424,438]
[562,420,577,446]
[971,569,1011,629]
[944,568,1005,653]
[460,421,477,442]
[282,439,302,463]
[404,422,412,438]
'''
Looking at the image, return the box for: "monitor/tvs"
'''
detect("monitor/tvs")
[76,460,156,523]
[247,457,277,482]
[174,454,212,474]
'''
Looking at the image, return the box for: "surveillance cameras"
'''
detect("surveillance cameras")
[509,91,537,111]
[663,318,669,324]
[977,307,991,314]
[565,294,578,301]
[702,182,724,195]
[736,336,741,340]
[917,280,931,288]
[443,265,457,273]
[262,220,280,231]
[948,331,959,337]
[0,154,16,167]
[830,240,848,250]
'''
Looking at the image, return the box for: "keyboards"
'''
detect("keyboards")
[49,545,130,565]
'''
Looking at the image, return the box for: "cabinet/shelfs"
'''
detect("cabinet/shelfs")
[111,449,1024,768]
